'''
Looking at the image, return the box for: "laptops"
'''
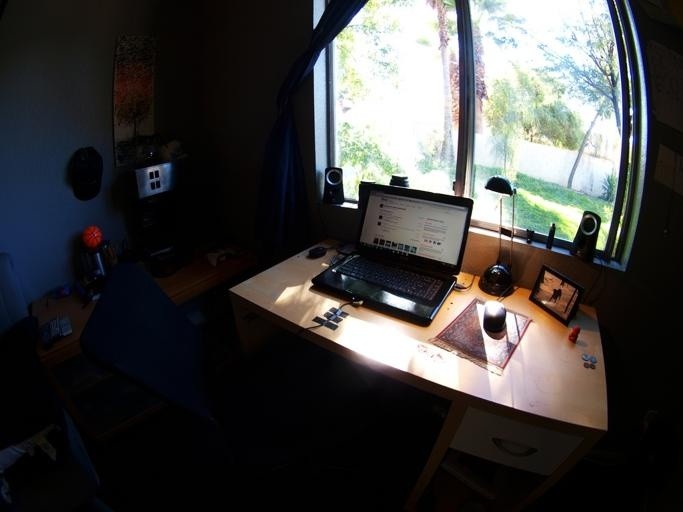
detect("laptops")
[311,180,474,326]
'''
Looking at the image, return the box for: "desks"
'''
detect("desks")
[226,237,609,509]
[28,241,267,451]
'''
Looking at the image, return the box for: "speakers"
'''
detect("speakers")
[323,167,344,204]
[569,210,603,264]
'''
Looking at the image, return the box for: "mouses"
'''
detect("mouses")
[482,299,507,332]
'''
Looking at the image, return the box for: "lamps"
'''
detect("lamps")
[479,175,518,298]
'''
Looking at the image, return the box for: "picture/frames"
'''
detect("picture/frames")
[528,265,585,328]
